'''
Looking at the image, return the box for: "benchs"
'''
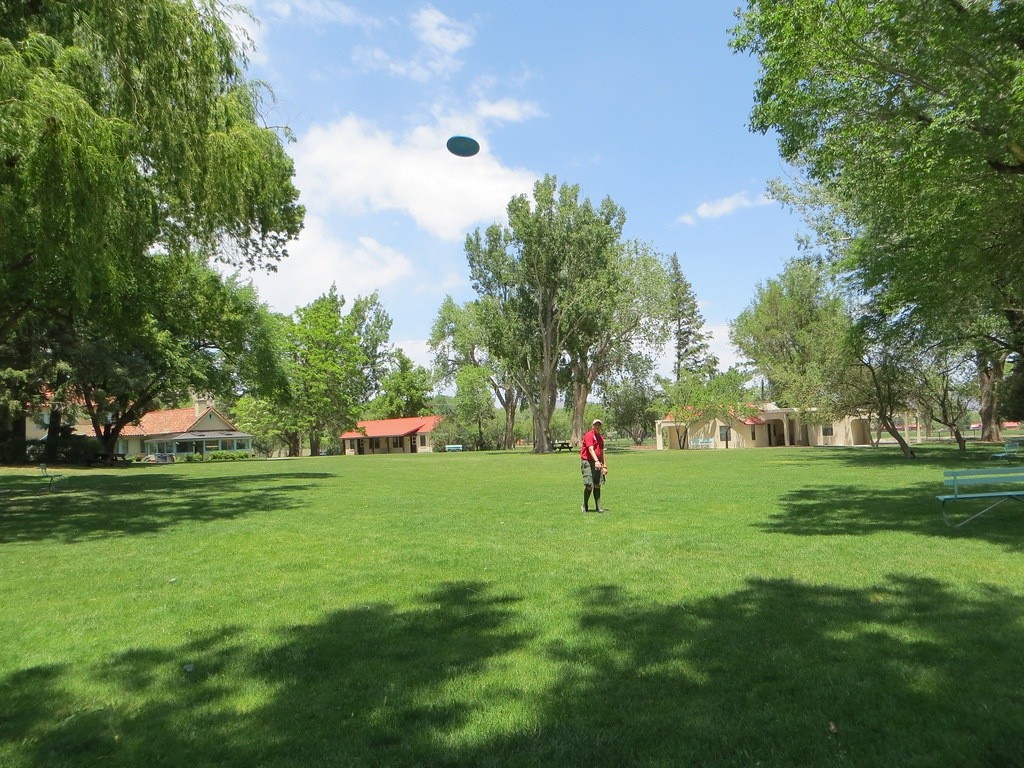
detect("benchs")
[988,443,1019,464]
[934,467,1024,528]
[110,459,133,468]
[87,460,107,467]
[558,447,573,451]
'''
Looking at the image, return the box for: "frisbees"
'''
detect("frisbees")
[447,133,481,158]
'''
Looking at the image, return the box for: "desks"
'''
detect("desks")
[558,443,570,447]
[109,453,128,460]
[86,453,108,459]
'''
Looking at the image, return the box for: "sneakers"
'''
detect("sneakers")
[596,509,604,513]
[581,505,588,513]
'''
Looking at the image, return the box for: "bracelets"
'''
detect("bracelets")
[603,467,607,469]
[595,460,599,462]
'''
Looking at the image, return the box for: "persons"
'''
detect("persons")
[580,419,608,513]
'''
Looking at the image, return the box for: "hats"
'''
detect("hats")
[593,419,602,425]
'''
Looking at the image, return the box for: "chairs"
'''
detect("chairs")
[39,463,66,491]
